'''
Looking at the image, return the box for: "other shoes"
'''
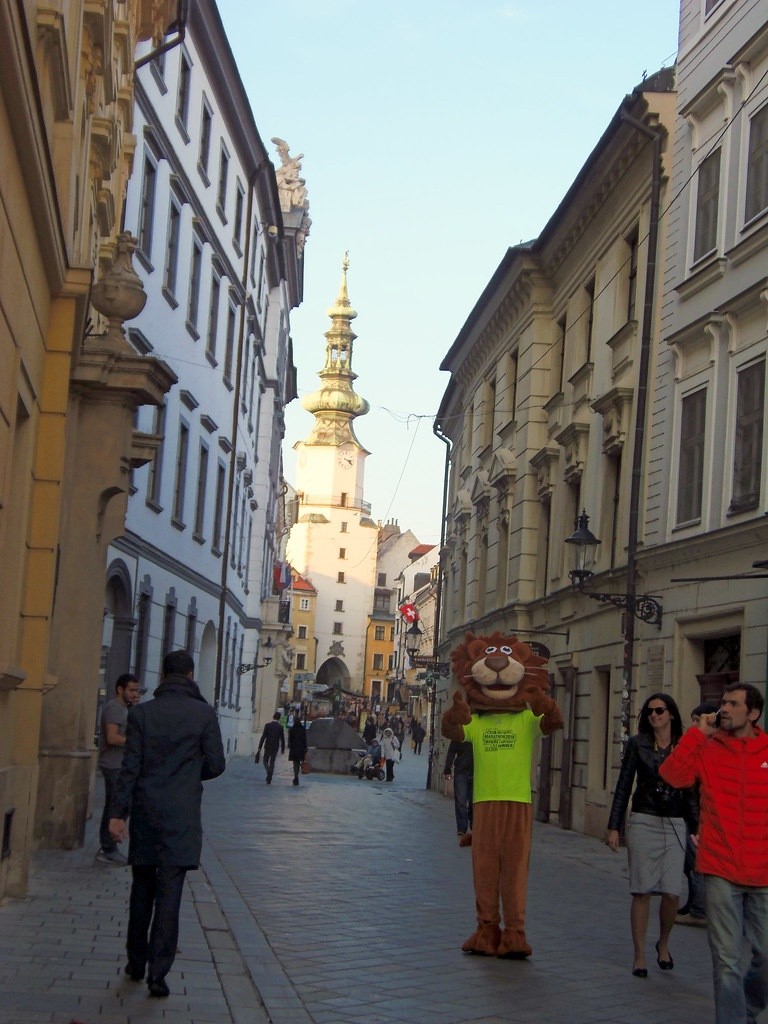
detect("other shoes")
[125,959,144,981]
[673,913,710,927]
[148,979,171,998]
[457,831,464,835]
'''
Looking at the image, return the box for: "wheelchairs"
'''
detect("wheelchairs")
[356,752,386,781]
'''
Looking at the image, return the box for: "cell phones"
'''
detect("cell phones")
[714,709,722,728]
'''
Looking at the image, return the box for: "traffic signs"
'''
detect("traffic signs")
[297,683,329,692]
[293,673,316,681]
[305,693,313,701]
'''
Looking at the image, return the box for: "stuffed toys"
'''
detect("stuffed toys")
[440,629,564,957]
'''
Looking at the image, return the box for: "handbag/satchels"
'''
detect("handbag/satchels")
[254,750,260,764]
[392,750,400,763]
[683,837,695,873]
[409,737,417,749]
[300,759,311,775]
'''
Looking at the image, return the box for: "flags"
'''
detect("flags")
[400,604,420,623]
[275,555,291,591]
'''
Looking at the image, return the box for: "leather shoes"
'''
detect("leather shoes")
[632,960,648,978]
[655,941,674,970]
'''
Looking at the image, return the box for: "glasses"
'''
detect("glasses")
[644,706,670,715]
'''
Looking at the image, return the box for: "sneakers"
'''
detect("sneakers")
[95,845,129,866]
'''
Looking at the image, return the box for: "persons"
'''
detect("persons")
[258,712,285,784]
[107,652,225,1000]
[660,678,768,1024]
[352,737,381,773]
[673,702,721,928]
[442,738,474,837]
[95,671,140,865]
[288,717,308,786]
[335,693,426,760]
[283,698,305,721]
[607,692,701,978]
[380,727,400,782]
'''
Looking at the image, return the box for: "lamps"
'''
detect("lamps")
[260,221,277,238]
[237,635,273,675]
[564,508,664,633]
[402,619,451,679]
